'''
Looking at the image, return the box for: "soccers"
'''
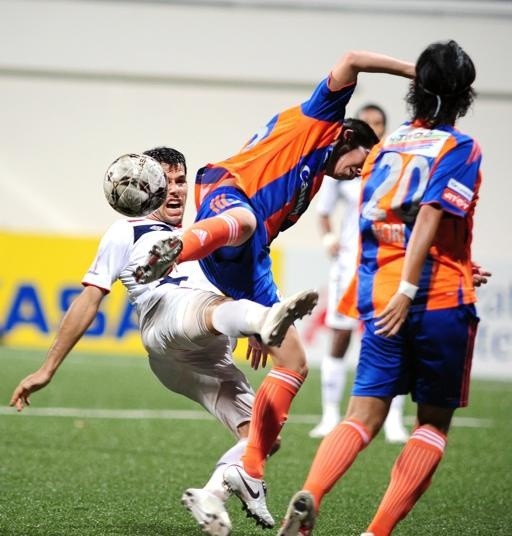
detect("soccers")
[104,153,168,217]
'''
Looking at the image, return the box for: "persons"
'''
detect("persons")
[132,49,416,528]
[9,147,318,536]
[276,40,492,536]
[310,104,409,443]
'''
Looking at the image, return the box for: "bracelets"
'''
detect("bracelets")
[397,279,419,300]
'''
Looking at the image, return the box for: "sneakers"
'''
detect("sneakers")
[360,532,374,536]
[383,408,410,443]
[223,461,275,530]
[181,487,233,536]
[260,289,319,348]
[131,237,184,285]
[275,490,316,536]
[309,415,343,438]
